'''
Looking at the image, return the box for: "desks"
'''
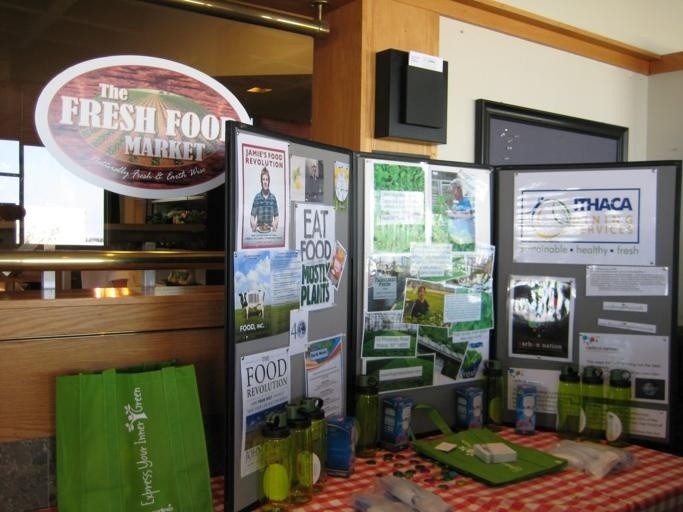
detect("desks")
[210,420,683,512]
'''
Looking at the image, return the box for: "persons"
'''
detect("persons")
[330,247,345,277]
[304,158,322,201]
[249,167,280,233]
[409,285,429,318]
[443,178,474,244]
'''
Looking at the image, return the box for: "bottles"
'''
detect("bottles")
[256,395,330,512]
[553,363,636,448]
[353,374,379,458]
[481,359,506,430]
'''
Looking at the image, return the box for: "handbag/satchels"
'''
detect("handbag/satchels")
[55,364,214,512]
[413,427,567,486]
